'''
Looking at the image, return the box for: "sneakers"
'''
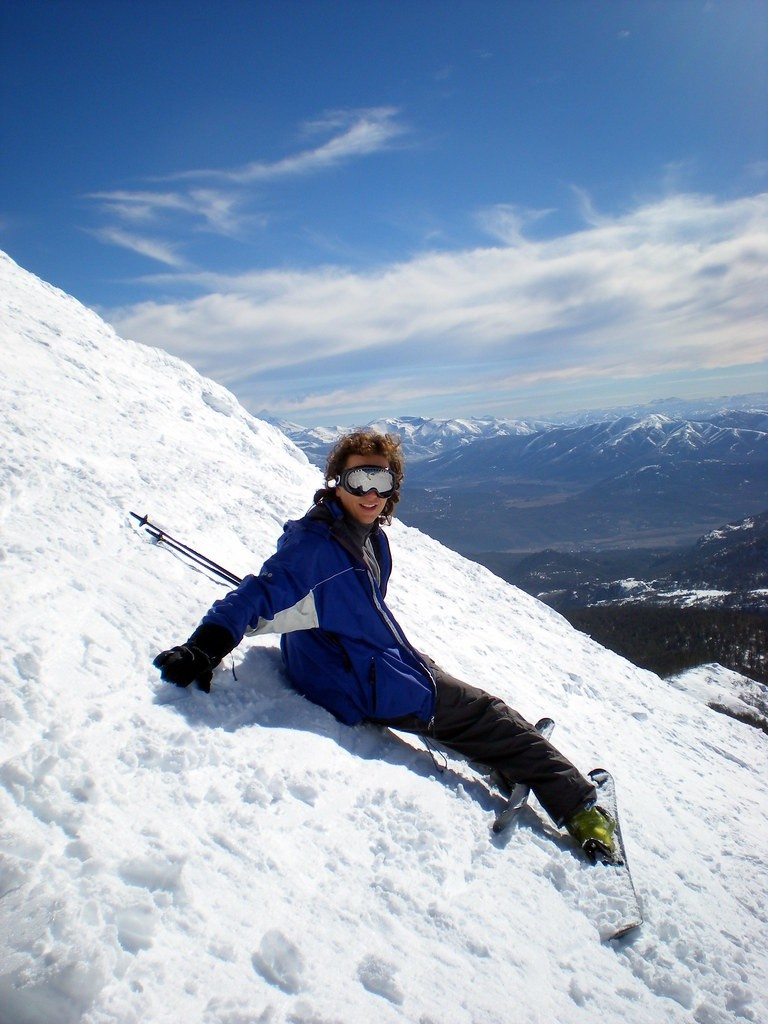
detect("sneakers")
[571,805,615,851]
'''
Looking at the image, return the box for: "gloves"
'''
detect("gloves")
[153,641,214,692]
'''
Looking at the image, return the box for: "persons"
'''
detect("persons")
[153,431,615,858]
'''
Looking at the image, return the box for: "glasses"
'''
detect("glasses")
[336,465,395,498]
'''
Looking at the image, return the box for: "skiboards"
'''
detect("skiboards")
[491,716,642,941]
